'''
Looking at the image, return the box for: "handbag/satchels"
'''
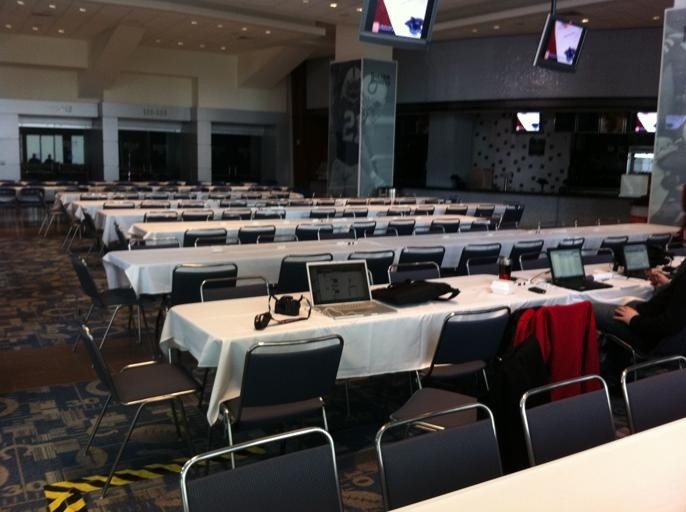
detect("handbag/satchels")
[370,279,459,304]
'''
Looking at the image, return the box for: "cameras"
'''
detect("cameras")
[274,295,301,316]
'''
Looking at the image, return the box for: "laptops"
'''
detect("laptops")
[621,242,652,280]
[306,259,400,322]
[547,245,613,292]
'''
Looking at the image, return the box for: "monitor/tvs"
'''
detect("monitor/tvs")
[357,0,440,54]
[634,111,657,133]
[533,13,589,74]
[630,152,654,174]
[513,111,544,134]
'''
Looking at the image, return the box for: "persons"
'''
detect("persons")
[27,152,39,162]
[591,181,686,379]
[43,153,58,166]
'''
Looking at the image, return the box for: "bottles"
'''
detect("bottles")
[498,255,512,280]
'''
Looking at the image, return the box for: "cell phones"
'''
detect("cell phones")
[528,287,546,294]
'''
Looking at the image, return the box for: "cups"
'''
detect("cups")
[492,280,514,295]
